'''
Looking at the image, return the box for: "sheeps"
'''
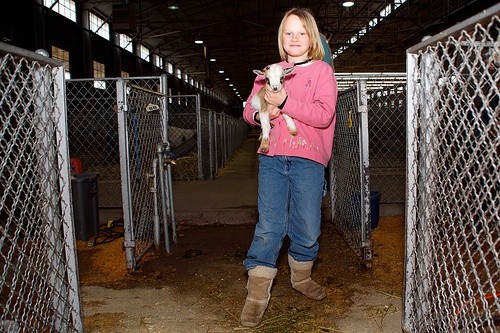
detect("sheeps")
[250,63,298,153]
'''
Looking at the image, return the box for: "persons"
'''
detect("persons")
[225,7,339,327]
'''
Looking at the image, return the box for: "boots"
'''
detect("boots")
[288,255,326,301]
[241,265,277,327]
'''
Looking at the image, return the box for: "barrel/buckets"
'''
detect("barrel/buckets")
[69,172,102,241]
[351,189,383,231]
